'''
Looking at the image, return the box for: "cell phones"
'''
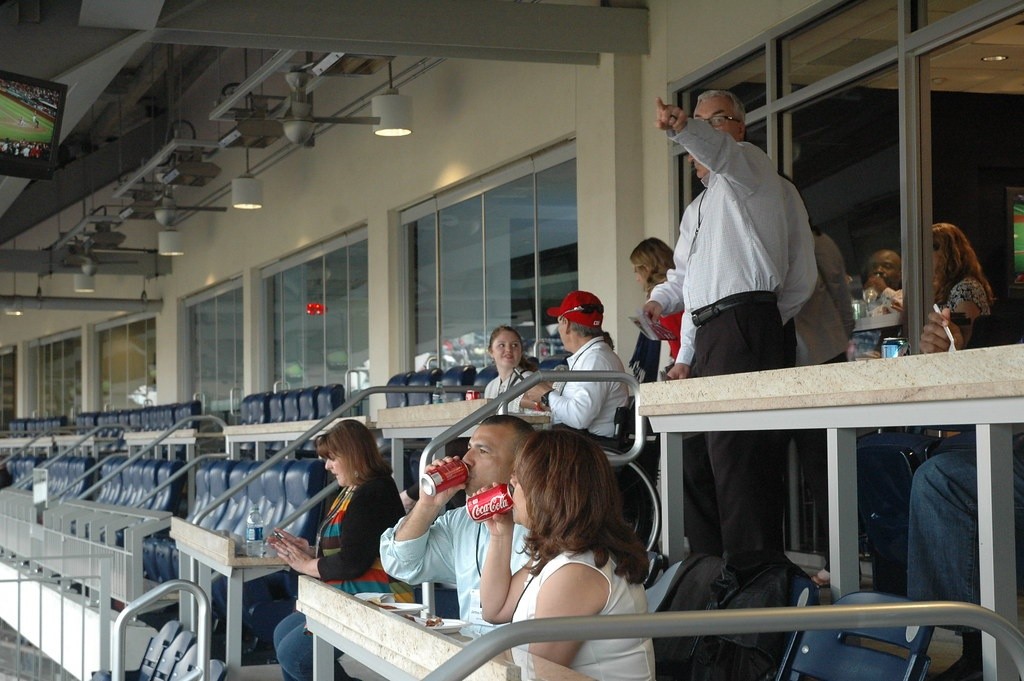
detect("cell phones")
[274,530,282,540]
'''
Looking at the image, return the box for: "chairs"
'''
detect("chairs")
[5,355,934,681]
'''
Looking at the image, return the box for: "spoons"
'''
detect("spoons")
[933,304,956,352]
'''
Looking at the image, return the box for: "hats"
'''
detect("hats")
[546,290,603,327]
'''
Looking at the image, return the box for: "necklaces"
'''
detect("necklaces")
[345,491,350,494]
[511,575,535,623]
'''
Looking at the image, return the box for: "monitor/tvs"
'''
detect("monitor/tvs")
[0,69,68,181]
[1005,187,1024,289]
[318,346,348,370]
[284,359,303,382]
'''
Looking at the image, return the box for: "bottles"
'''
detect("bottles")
[246,506,264,557]
[863,273,880,302]
[433,382,447,404]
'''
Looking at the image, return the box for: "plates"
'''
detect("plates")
[413,616,471,634]
[378,602,427,615]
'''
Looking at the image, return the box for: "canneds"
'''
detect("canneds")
[880,336,910,358]
[465,389,480,401]
[851,300,866,319]
[466,483,515,524]
[421,459,469,497]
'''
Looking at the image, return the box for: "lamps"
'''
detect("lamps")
[372,56,413,136]
[158,226,185,257]
[73,274,96,293]
[231,147,263,209]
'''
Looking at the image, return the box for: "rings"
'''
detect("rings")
[286,551,289,556]
[670,114,677,119]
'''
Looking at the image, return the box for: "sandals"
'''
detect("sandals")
[808,574,862,588]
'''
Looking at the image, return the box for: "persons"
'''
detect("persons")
[483,291,629,439]
[480,428,655,681]
[906,282,1024,612]
[380,415,535,634]
[666,90,790,556]
[266,419,415,681]
[0,79,59,160]
[629,154,994,437]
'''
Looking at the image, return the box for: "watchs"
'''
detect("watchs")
[541,388,556,408]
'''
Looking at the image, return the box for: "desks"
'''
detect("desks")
[375,399,551,493]
[223,416,376,462]
[123,428,225,513]
[636,338,1024,681]
[54,435,119,500]
[166,517,291,680]
[0,437,53,460]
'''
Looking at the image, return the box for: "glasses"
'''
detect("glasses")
[933,243,940,251]
[696,115,741,131]
[559,303,603,321]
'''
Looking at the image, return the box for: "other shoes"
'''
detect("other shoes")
[930,631,984,681]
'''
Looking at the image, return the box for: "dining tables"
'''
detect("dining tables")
[296,575,599,681]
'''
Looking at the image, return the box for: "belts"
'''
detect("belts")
[692,293,778,327]
